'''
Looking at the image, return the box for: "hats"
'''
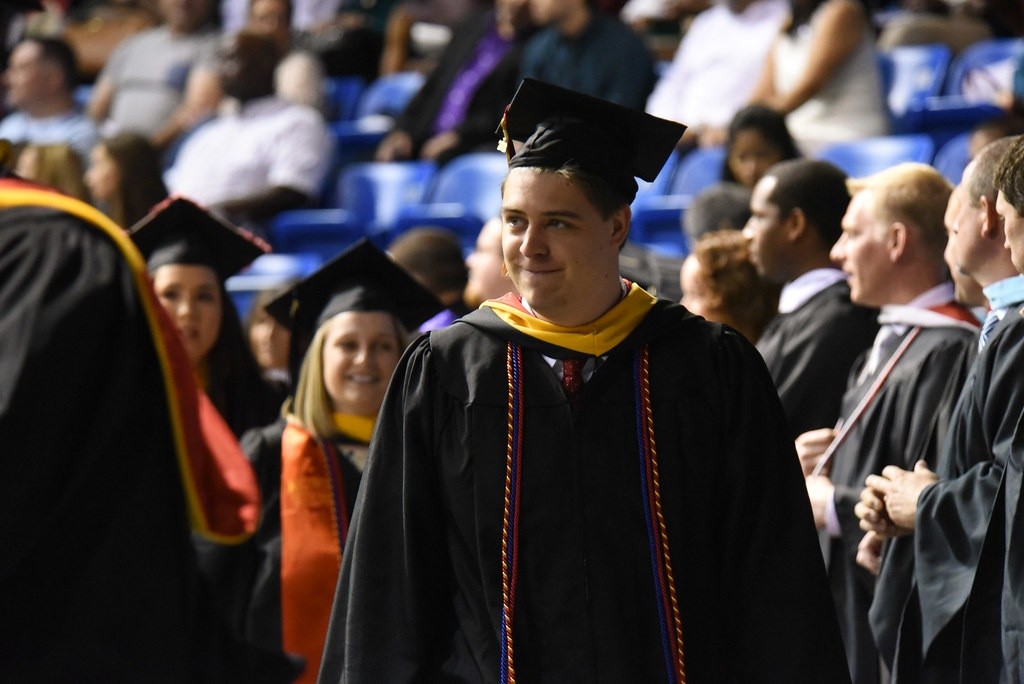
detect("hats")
[263,236,449,334]
[124,197,271,283]
[495,77,687,205]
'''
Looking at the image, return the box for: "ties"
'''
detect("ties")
[558,355,591,401]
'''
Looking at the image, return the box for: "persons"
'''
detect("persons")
[317,78,850,684]
[742,158,883,441]
[0,0,660,318]
[795,163,984,684]
[0,165,289,684]
[242,238,448,684]
[646,0,1024,251]
[678,230,780,346]
[854,135,1024,684]
